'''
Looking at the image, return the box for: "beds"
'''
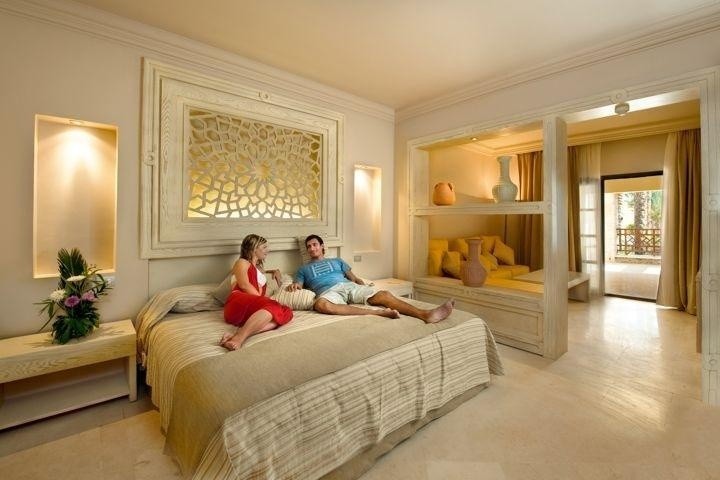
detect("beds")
[134,284,504,480]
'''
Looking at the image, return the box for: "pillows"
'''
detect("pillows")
[296,232,328,264]
[480,249,497,272]
[213,271,233,305]
[269,275,316,311]
[441,250,461,279]
[493,239,514,266]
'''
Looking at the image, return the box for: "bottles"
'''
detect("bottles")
[432,181,455,206]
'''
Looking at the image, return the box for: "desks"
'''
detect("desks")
[0,319,137,430]
[513,268,590,304]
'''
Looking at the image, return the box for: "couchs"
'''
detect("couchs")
[428,236,544,294]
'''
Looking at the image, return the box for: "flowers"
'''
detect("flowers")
[34,248,111,345]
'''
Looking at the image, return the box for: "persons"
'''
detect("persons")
[219,234,293,351]
[285,235,455,324]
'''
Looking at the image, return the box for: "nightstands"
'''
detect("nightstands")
[373,277,413,300]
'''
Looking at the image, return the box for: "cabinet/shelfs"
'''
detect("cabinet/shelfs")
[408,118,543,355]
